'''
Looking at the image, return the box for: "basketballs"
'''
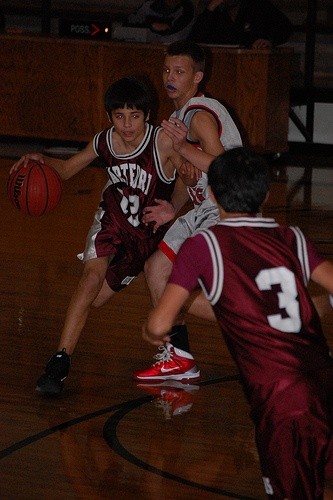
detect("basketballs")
[7,163,63,217]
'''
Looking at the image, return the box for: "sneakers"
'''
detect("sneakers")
[136,383,200,420]
[35,347,71,394]
[132,342,201,383]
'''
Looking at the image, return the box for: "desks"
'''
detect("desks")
[0,36,296,155]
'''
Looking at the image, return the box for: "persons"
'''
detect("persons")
[132,40,332,382]
[111,0,198,43]
[9,78,203,395]
[142,147,332,500]
[193,0,293,49]
